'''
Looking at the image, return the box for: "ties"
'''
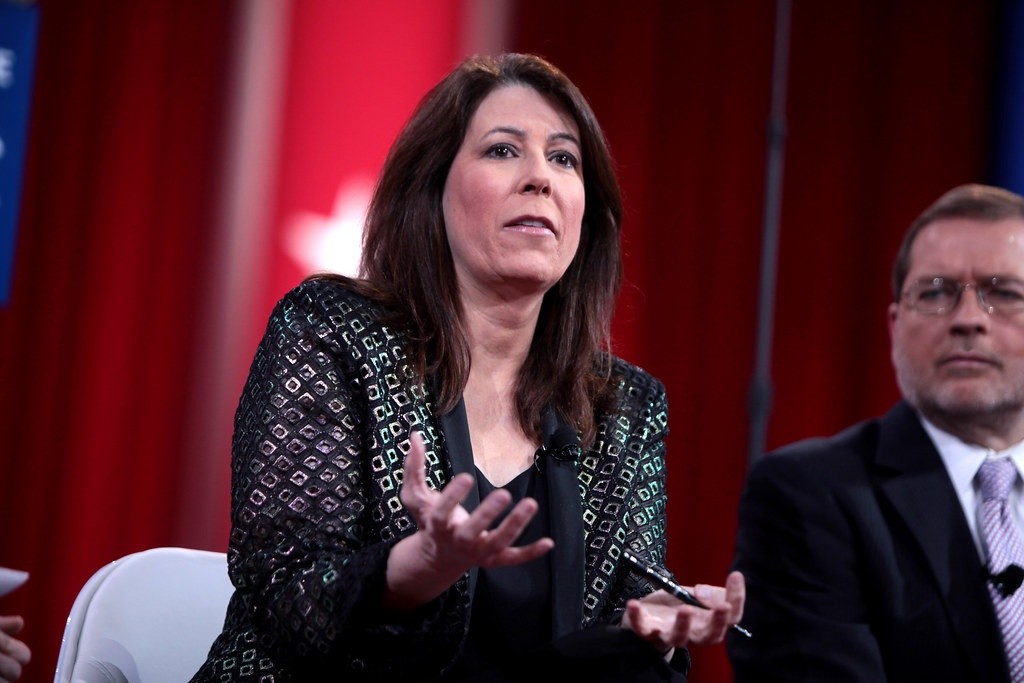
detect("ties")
[976,458,1023,683]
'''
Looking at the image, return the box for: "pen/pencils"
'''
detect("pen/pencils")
[621,552,752,640]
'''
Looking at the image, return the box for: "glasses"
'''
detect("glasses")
[902,273,1023,317]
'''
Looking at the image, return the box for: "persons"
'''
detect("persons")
[723,185,1024,683]
[1,614,31,683]
[190,51,746,683]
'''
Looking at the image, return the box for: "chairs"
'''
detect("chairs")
[53,546,236,683]
